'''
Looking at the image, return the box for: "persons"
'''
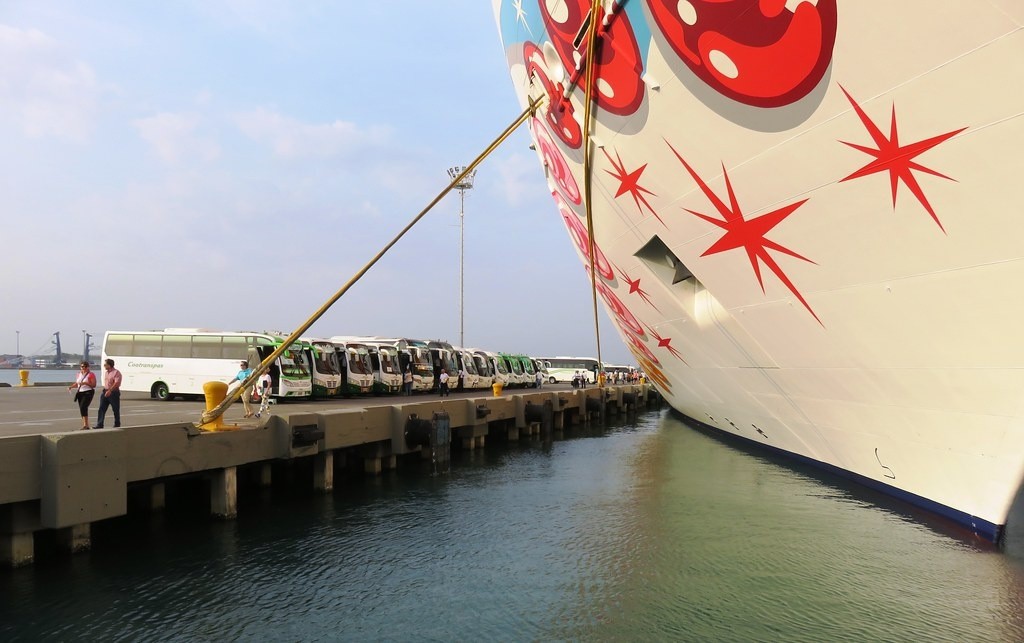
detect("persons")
[229,360,257,418]
[68,362,97,430]
[439,369,450,397]
[458,369,463,385]
[573,370,580,388]
[581,371,586,388]
[92,359,122,429]
[404,369,413,396]
[612,370,650,385]
[536,369,542,389]
[607,371,610,383]
[255,365,272,418]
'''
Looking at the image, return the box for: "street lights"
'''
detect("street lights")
[16,330,21,356]
[448,164,478,348]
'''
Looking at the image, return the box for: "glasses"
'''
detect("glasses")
[80,366,87,370]
[240,364,244,366]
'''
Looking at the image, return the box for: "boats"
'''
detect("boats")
[490,1,1024,551]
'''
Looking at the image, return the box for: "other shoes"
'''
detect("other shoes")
[255,413,263,418]
[113,424,120,427]
[92,425,104,429]
[79,426,90,430]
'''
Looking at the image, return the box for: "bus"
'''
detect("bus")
[100,328,319,404]
[330,334,552,400]
[537,356,605,385]
[329,339,376,398]
[604,365,637,382]
[279,333,343,403]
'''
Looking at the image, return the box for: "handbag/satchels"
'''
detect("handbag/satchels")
[252,390,260,401]
[74,391,78,401]
